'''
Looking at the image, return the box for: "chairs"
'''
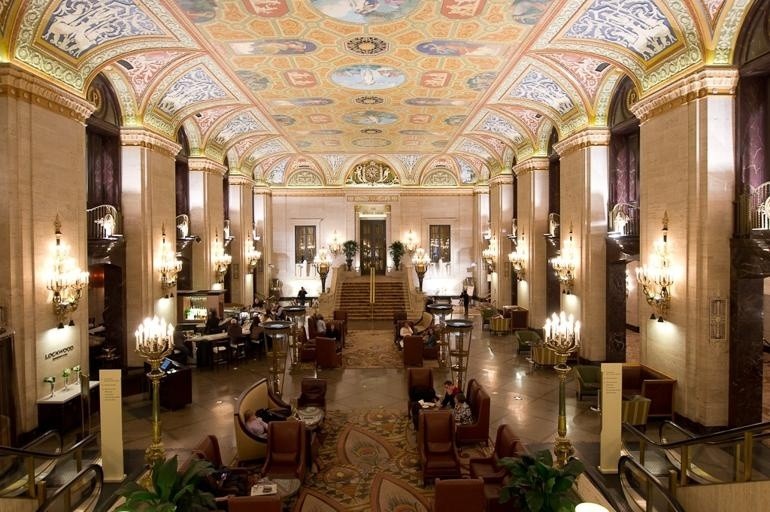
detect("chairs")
[392,311,408,327]
[313,335,342,369]
[414,311,436,335]
[423,328,442,360]
[264,420,311,483]
[393,321,416,345]
[326,319,349,347]
[406,368,440,418]
[298,377,329,418]
[295,326,315,364]
[432,476,484,512]
[472,425,530,509]
[307,315,325,340]
[402,337,425,373]
[479,306,652,433]
[227,494,283,512]
[332,310,349,332]
[460,378,490,447]
[416,408,460,487]
[177,432,245,511]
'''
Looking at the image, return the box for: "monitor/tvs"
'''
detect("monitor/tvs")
[161,359,171,370]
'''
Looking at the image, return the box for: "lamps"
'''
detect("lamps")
[542,311,610,464]
[313,228,431,293]
[46,213,261,329]
[134,315,174,465]
[482,210,674,323]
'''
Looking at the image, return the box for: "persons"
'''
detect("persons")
[205,310,219,331]
[460,290,470,315]
[245,410,269,439]
[453,392,474,429]
[425,328,436,346]
[400,322,413,337]
[298,287,307,307]
[317,316,327,334]
[441,380,464,409]
[228,298,286,355]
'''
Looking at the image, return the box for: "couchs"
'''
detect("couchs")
[231,377,292,462]
